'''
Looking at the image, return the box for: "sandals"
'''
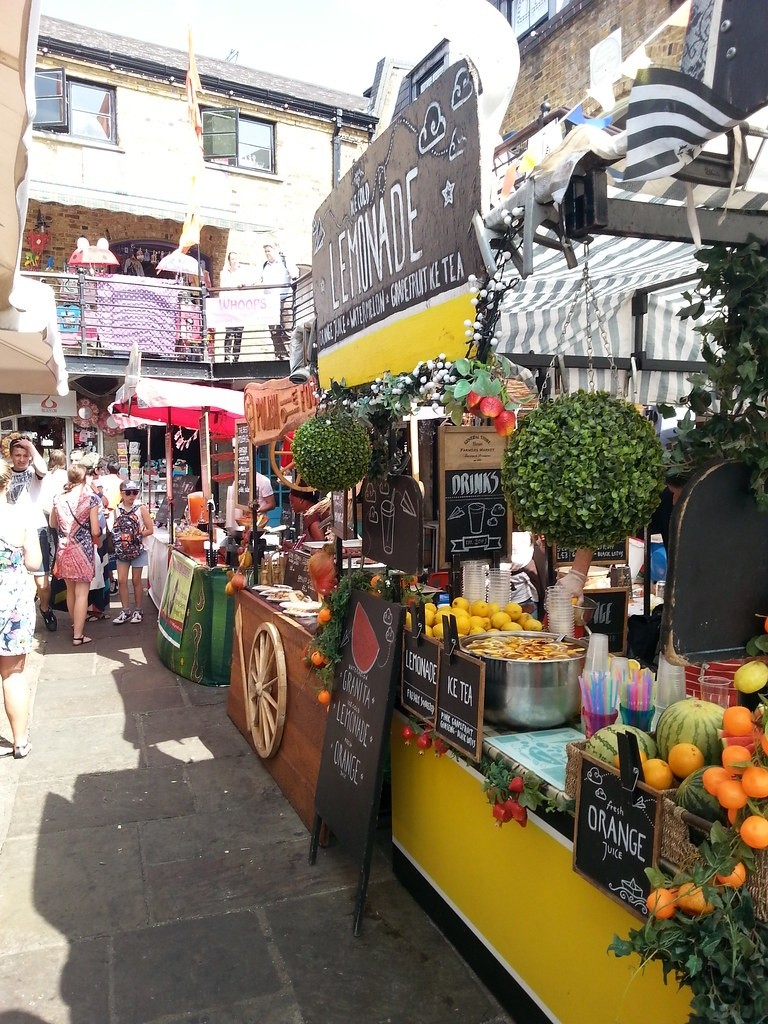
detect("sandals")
[73,635,93,645]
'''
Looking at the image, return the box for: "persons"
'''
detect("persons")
[0,430,124,759]
[511,532,594,621]
[289,479,330,542]
[220,252,253,363]
[251,245,299,361]
[256,472,276,512]
[113,481,153,623]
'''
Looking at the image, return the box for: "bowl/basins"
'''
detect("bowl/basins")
[237,517,255,528]
[180,537,209,557]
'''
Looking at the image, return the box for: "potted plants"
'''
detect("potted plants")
[177,526,209,551]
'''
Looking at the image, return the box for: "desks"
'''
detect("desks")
[146,526,694,1024]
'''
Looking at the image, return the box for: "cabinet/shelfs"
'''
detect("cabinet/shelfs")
[141,464,188,511]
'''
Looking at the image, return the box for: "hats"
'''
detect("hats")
[120,480,141,490]
[79,452,104,469]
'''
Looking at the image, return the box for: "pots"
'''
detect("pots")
[460,630,589,729]
[221,515,270,542]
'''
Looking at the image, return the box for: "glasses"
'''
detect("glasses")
[124,491,139,496]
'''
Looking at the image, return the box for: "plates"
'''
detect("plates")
[252,585,321,618]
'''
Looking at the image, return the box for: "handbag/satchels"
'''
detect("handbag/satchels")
[89,545,105,590]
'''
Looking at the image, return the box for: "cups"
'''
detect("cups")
[582,707,618,742]
[226,481,243,529]
[461,560,577,638]
[620,705,655,734]
[580,632,630,679]
[656,651,731,709]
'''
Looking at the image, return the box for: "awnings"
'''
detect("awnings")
[0,0,40,313]
[0,275,69,396]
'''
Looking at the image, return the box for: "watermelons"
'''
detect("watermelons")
[657,700,726,765]
[585,724,657,769]
[675,765,728,826]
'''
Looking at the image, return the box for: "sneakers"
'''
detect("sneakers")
[109,579,119,596]
[130,611,143,623]
[39,603,57,632]
[113,610,131,624]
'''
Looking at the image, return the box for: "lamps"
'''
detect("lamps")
[33,208,51,234]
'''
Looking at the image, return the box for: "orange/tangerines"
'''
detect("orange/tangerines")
[371,575,382,598]
[647,706,768,919]
[610,742,704,791]
[402,576,418,605]
[310,608,330,703]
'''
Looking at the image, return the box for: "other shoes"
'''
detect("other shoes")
[0,740,32,758]
[85,615,98,621]
[94,612,111,619]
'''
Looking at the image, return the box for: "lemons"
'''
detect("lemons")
[572,597,578,605]
[733,661,768,693]
[406,598,542,638]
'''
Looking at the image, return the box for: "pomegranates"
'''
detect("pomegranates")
[402,724,448,758]
[493,803,512,829]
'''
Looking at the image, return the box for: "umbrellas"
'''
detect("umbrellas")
[155,249,204,276]
[69,238,120,273]
[107,377,244,435]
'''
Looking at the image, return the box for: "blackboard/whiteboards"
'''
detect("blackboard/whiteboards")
[440,468,514,565]
[582,588,630,655]
[399,627,485,762]
[155,474,200,527]
[234,419,254,511]
[314,588,405,863]
[165,432,172,500]
[362,476,422,578]
[576,756,661,920]
[198,412,211,500]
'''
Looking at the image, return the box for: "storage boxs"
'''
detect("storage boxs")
[301,539,362,558]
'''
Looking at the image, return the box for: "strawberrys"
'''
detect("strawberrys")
[503,777,528,828]
[467,390,515,436]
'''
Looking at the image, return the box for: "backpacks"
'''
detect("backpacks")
[112,503,146,562]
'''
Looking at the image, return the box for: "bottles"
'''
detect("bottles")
[203,536,294,585]
[171,505,208,545]
